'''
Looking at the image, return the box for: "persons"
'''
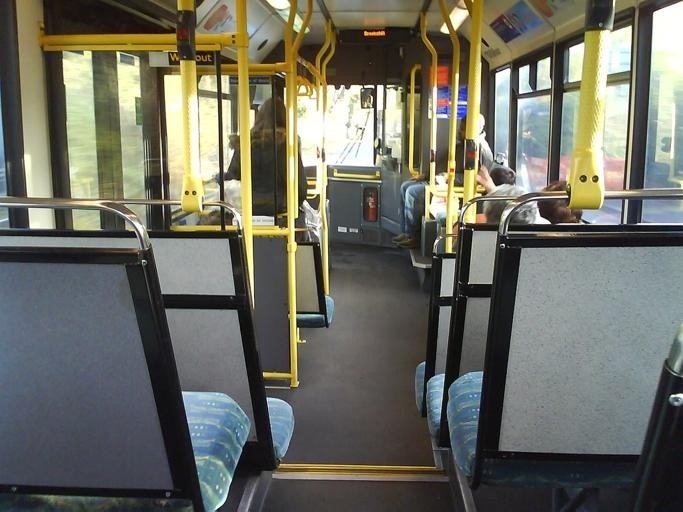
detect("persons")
[534,174,591,225]
[484,160,516,192]
[393,114,494,247]
[464,182,538,234]
[224,94,326,261]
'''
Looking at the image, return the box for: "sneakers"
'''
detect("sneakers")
[397,238,416,249]
[391,233,407,243]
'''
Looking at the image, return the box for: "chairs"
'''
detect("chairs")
[0,197,251,512]
[418,152,505,255]
[112,197,296,470]
[448,188,683,511]
[425,195,520,467]
[415,234,458,418]
[296,228,333,328]
[630,332,682,512]
